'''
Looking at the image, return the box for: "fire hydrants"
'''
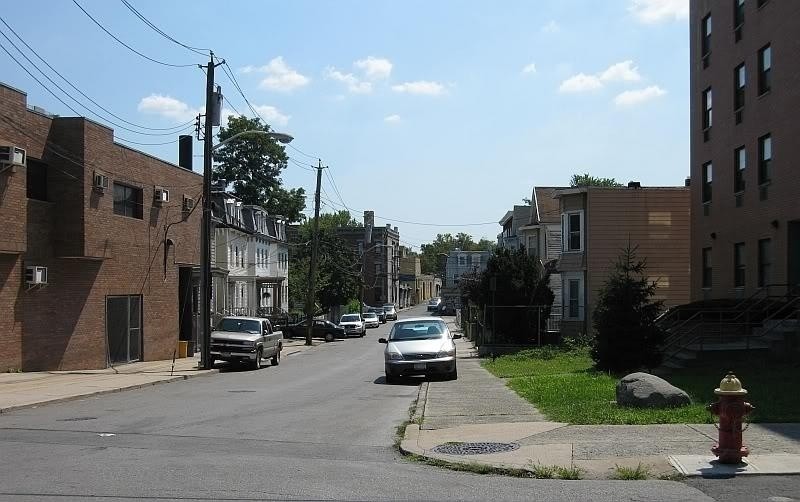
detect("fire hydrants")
[706,371,755,466]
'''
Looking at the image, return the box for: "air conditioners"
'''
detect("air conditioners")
[0,145,27,167]
[25,265,48,287]
[155,188,170,203]
[96,174,109,189]
[185,198,194,210]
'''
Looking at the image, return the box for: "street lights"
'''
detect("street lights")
[360,243,394,318]
[200,130,295,369]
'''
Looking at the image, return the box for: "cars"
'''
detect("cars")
[339,313,366,337]
[378,317,462,380]
[280,317,346,343]
[427,297,441,311]
[361,302,399,328]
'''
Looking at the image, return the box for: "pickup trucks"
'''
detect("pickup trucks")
[209,314,284,369]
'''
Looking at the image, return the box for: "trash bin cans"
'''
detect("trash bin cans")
[179,341,195,358]
